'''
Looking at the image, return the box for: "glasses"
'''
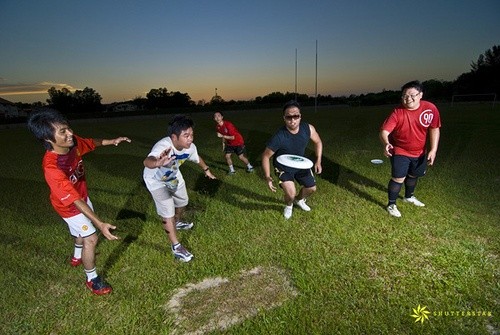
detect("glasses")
[284,115,301,121]
[402,92,422,100]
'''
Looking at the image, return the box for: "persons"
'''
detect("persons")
[143,115,217,263]
[378,80,441,218]
[261,100,324,220]
[29,110,131,296]
[214,111,254,175]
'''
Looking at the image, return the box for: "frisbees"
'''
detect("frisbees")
[371,159,384,164]
[276,154,313,169]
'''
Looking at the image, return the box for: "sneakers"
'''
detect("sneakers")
[171,242,195,263]
[246,166,254,172]
[71,257,83,268]
[386,204,401,218]
[284,203,293,219]
[402,195,426,207]
[227,171,236,175]
[294,198,312,212]
[85,275,113,296]
[175,220,194,230]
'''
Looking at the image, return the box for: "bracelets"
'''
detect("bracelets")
[204,168,209,171]
